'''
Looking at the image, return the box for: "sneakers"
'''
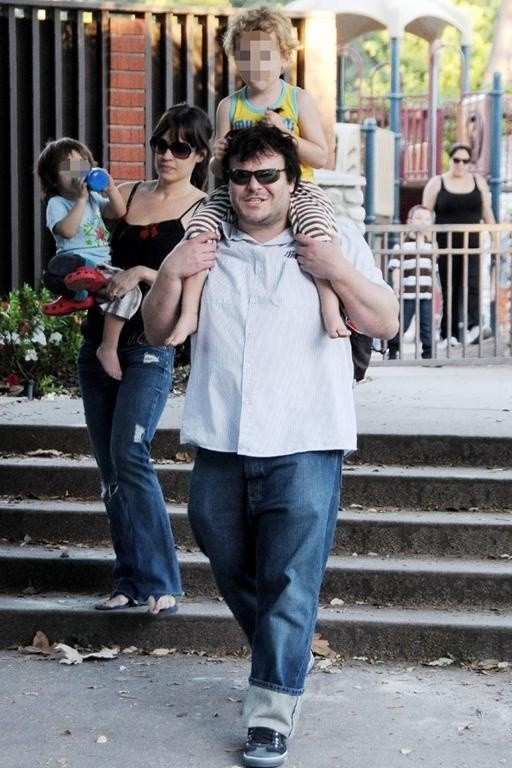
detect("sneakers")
[443,325,482,349]
[239,720,289,768]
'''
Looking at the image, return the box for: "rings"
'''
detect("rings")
[110,290,116,295]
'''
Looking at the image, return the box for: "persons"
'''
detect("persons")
[161,4,357,347]
[420,142,502,349]
[385,202,441,359]
[40,102,215,622]
[34,137,144,381]
[139,120,402,768]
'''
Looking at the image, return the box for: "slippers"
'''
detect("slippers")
[93,591,136,611]
[145,593,179,618]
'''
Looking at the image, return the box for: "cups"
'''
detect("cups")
[76,169,109,192]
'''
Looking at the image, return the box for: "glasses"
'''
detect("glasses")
[225,165,290,186]
[451,156,471,165]
[149,136,198,160]
